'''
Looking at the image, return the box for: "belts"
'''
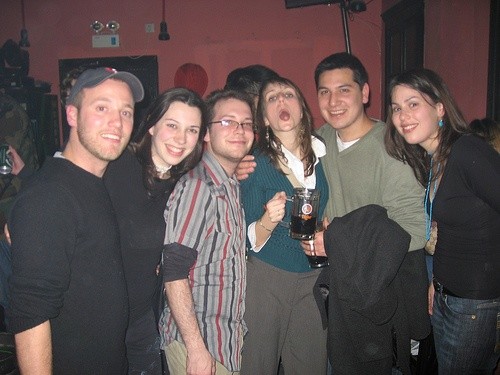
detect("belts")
[433,277,464,298]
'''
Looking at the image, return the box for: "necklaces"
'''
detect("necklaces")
[424,147,451,240]
[151,157,173,179]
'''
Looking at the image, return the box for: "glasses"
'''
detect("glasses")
[205,120,258,131]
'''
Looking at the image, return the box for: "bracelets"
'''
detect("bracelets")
[260,216,274,232]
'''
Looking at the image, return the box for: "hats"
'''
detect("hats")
[68,67,144,102]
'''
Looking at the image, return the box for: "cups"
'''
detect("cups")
[279,188,320,240]
[301,223,329,268]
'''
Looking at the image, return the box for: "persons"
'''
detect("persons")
[9,86,209,375]
[233,51,432,375]
[158,86,254,375]
[384,68,500,375]
[0,66,144,375]
[239,76,329,375]
[0,85,40,235]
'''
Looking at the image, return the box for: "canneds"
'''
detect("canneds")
[0,143,13,175]
